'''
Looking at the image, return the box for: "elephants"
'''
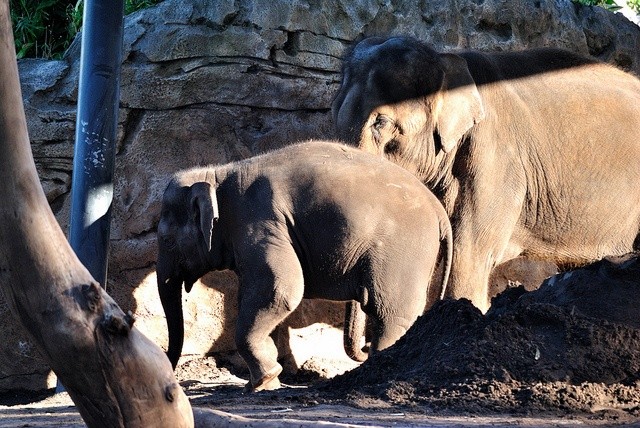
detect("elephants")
[330,34,640,363]
[154,137,454,395]
